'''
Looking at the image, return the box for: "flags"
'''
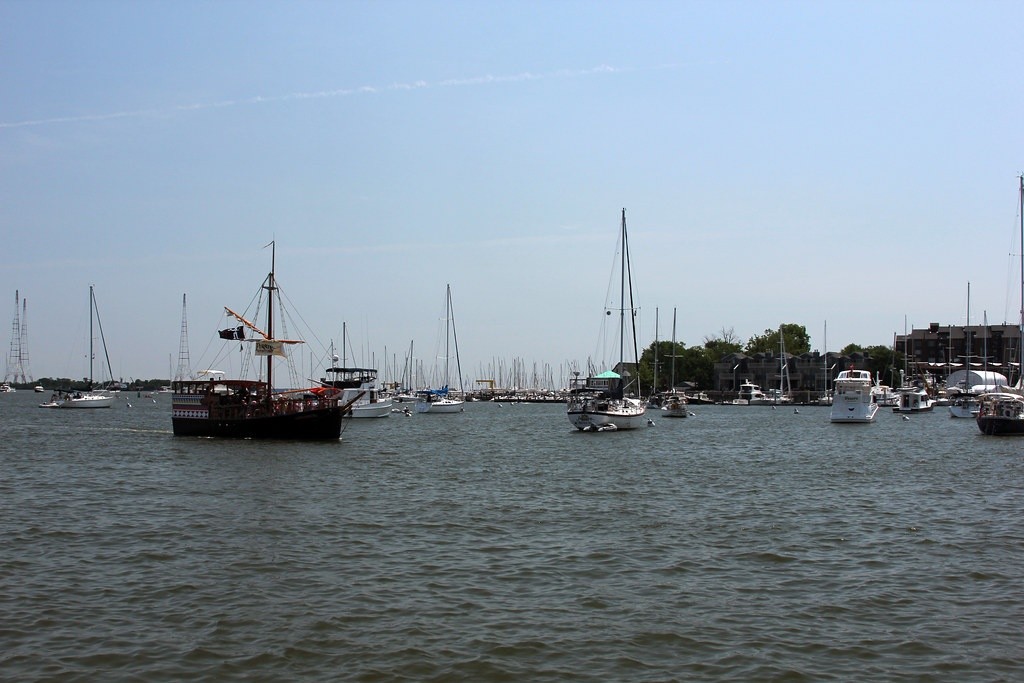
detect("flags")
[218,326,245,340]
[255,340,286,357]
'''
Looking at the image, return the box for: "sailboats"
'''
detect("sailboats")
[388,284,467,414]
[947,282,983,418]
[319,322,379,404]
[169,239,368,443]
[974,174,1024,435]
[39,284,120,409]
[660,308,687,417]
[381,341,568,403]
[567,208,647,431]
[817,320,833,406]
[647,308,665,408]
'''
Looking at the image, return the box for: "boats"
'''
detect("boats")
[34,386,45,392]
[685,392,714,403]
[0,384,12,393]
[830,364,880,423]
[870,387,900,406]
[892,387,937,414]
[195,369,233,394]
[738,325,795,406]
[305,379,393,418]
[934,395,976,405]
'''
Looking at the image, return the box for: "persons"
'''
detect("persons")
[59,391,63,397]
[406,412,411,416]
[403,407,409,413]
